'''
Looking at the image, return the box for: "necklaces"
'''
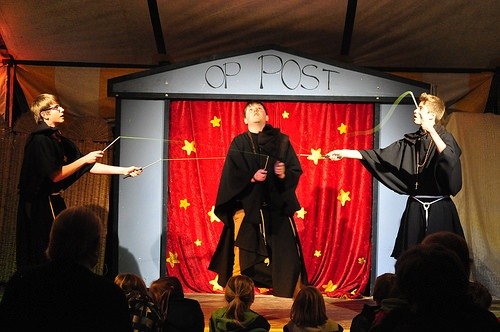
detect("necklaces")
[414,139,433,189]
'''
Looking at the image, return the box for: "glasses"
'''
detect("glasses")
[42,106,58,111]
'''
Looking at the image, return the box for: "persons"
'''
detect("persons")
[0,206,205,332]
[282,286,343,332]
[15,93,143,278]
[207,100,311,300]
[350,231,500,332]
[325,93,465,260]
[209,275,271,332]
